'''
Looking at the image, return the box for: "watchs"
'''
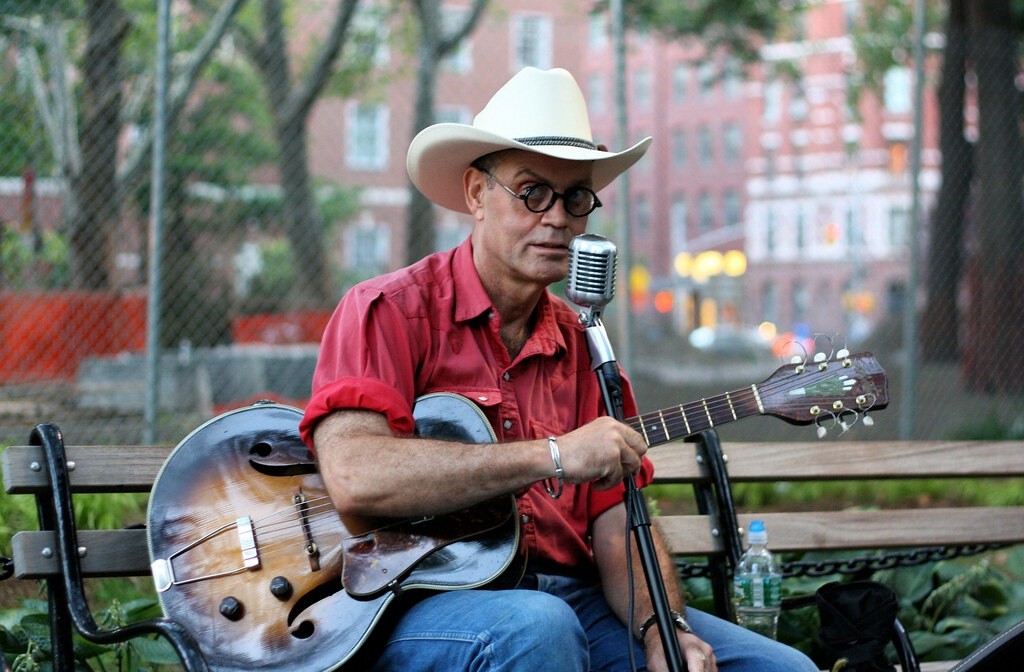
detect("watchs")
[638,610,692,650]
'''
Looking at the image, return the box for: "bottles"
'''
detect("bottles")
[733,521,781,642]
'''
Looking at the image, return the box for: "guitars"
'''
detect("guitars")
[145,332,891,672]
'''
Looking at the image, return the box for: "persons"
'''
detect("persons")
[299,65,821,672]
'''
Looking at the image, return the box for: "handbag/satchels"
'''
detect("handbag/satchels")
[809,582,898,672]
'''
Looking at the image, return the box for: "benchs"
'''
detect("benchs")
[0,422,1024,672]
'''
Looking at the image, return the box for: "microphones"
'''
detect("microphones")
[566,234,618,326]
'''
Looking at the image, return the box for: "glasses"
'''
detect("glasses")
[478,164,604,213]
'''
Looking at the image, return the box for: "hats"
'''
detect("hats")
[405,66,654,214]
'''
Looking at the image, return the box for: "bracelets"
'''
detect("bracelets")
[541,436,565,499]
